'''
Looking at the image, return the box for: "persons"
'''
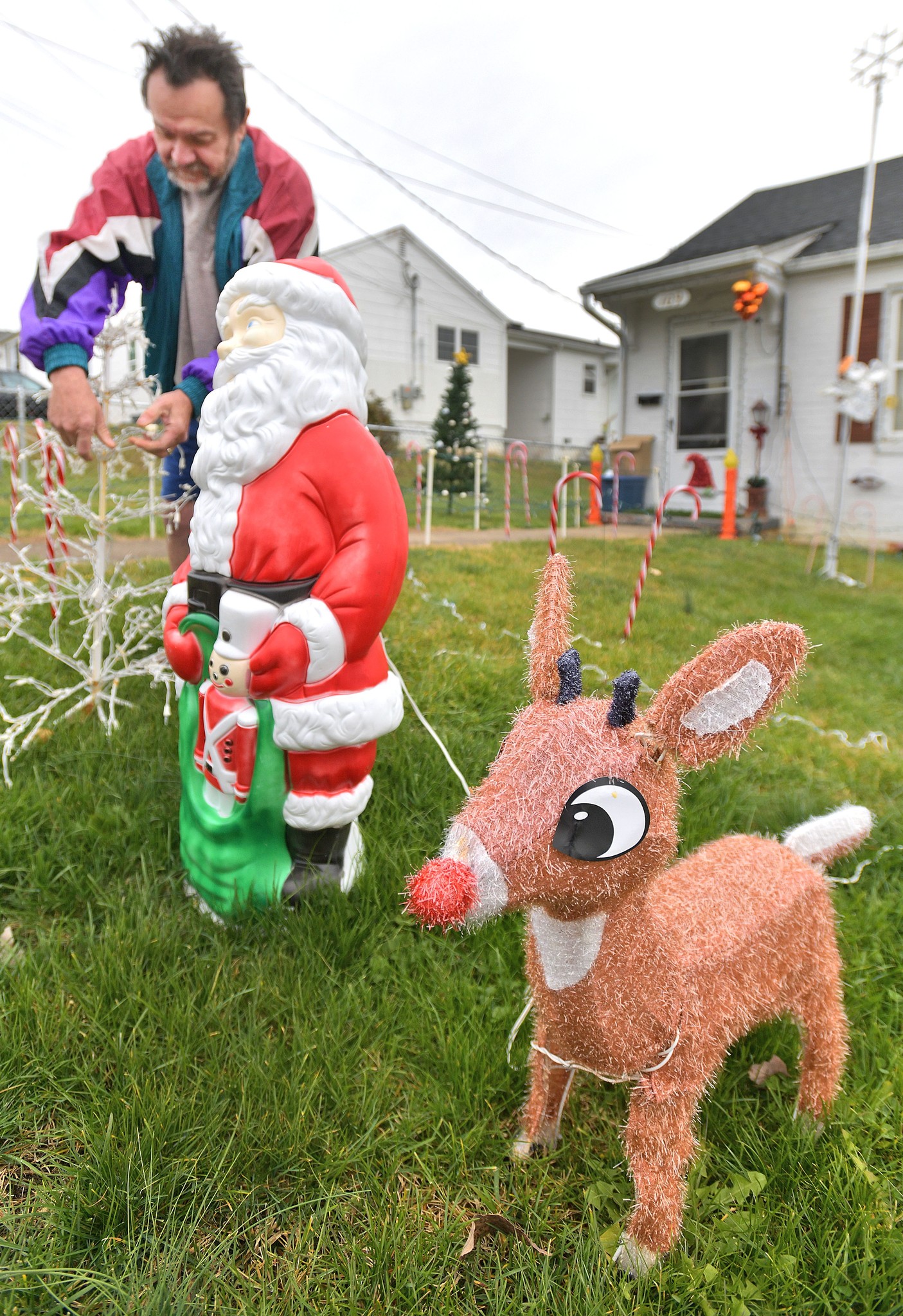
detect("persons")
[162,255,408,924]
[18,24,320,575]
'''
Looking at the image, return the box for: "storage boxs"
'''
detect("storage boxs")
[600,475,646,514]
